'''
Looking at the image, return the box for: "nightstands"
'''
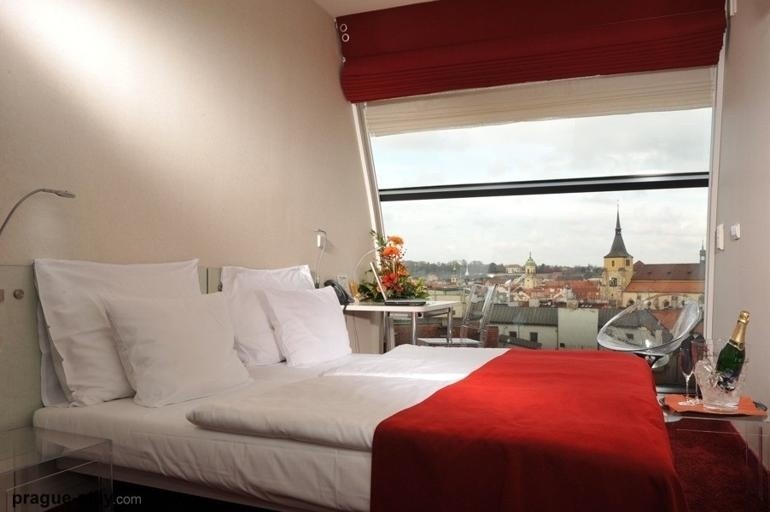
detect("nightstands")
[0,425,112,511]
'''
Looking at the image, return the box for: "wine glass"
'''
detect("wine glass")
[678,341,708,406]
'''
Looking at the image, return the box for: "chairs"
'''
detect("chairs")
[597,293,704,370]
[418,283,498,348]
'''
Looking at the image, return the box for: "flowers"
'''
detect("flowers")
[357,228,431,305]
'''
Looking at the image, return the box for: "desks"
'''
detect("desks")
[658,395,768,501]
[340,300,462,344]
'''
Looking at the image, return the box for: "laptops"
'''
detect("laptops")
[369,261,427,306]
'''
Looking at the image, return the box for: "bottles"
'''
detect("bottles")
[714,311,750,392]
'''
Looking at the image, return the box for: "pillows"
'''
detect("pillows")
[34,257,353,407]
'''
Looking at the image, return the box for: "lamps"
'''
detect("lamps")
[1,188,75,232]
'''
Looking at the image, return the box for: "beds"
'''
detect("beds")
[32,352,672,510]
